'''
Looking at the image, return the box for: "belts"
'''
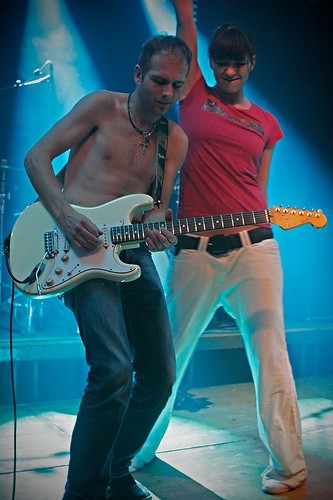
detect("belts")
[181,227,273,254]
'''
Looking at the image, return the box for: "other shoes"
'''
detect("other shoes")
[111,473,152,500]
[262,482,292,493]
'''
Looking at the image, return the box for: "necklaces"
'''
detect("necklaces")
[128,91,159,150]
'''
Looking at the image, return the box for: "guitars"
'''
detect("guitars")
[3,193,327,300]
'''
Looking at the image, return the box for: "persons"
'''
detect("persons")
[24,33,192,500]
[123,0,309,493]
